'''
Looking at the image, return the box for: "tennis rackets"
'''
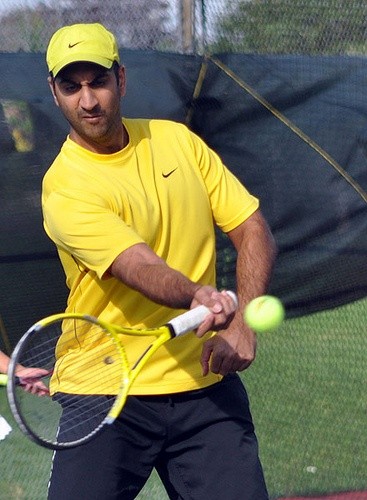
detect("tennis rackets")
[7,291,238,451]
[0,371,54,394]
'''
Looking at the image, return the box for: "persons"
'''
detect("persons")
[41,21,278,500]
[0,349,50,397]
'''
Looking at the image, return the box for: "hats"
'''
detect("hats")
[45,23,121,79]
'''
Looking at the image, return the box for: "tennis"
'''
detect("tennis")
[243,295,284,331]
